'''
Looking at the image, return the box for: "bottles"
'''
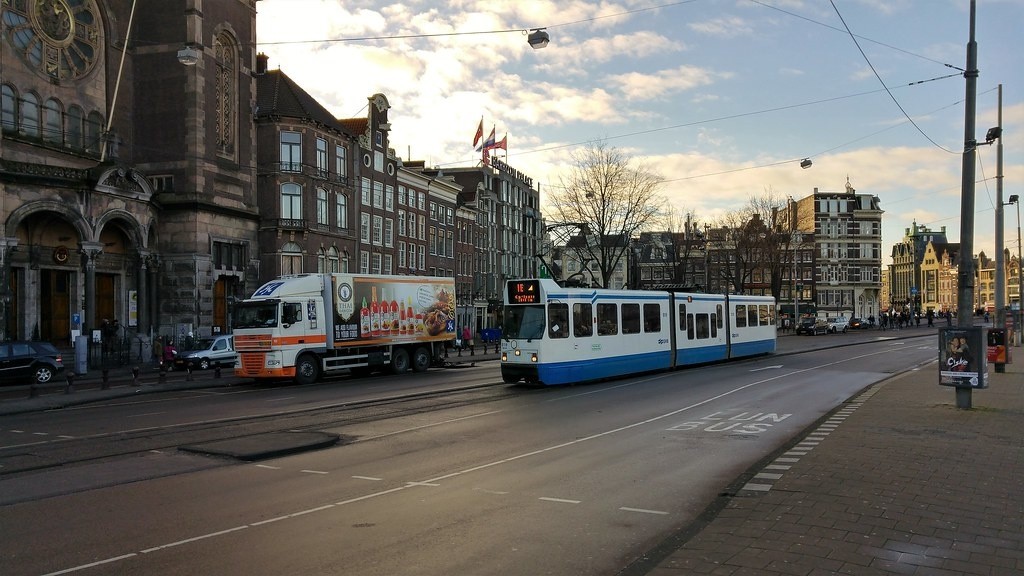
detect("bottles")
[360,286,423,339]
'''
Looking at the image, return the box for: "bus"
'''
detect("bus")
[499,222,777,386]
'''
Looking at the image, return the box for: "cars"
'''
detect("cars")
[849,318,869,330]
[173,334,236,371]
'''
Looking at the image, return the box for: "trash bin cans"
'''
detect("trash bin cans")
[481,328,501,341]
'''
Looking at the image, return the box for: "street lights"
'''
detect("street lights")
[953,126,1004,409]
[994,193,1021,373]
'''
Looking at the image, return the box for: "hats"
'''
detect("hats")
[188,332,193,336]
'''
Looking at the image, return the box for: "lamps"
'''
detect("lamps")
[800,158,813,169]
[528,30,549,49]
[586,192,594,199]
[177,46,198,66]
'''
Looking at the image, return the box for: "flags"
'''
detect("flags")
[476,127,495,151]
[488,136,507,151]
[473,119,483,146]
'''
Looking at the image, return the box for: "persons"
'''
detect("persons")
[184,331,196,374]
[462,325,472,350]
[162,339,177,368]
[948,337,971,372]
[781,305,990,329]
[154,334,162,367]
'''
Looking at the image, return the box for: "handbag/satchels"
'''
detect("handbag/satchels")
[469,339,474,346]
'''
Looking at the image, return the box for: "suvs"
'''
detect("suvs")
[826,316,850,333]
[0,340,66,384]
[795,316,829,336]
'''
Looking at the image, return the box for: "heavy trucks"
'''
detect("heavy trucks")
[232,271,458,384]
